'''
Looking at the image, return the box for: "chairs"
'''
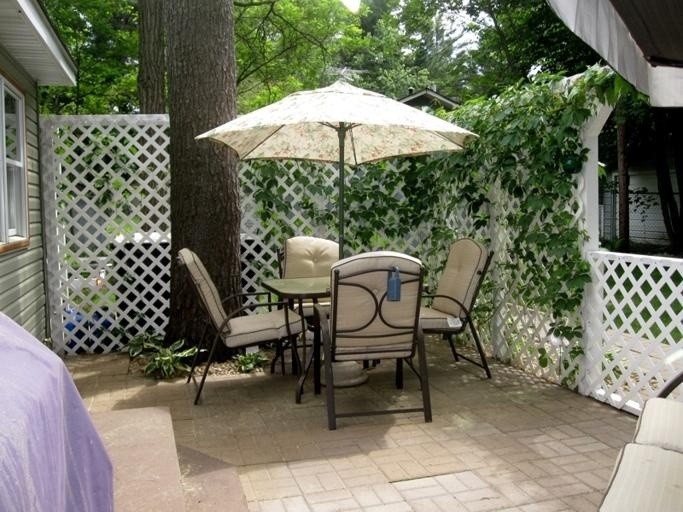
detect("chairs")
[394,238,496,388]
[270,236,340,376]
[314,252,433,429]
[176,249,307,406]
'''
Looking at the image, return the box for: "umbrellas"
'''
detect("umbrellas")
[191,76,480,259]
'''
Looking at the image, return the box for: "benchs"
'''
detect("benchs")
[596,370,683,512]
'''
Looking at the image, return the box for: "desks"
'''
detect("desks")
[260,274,429,396]
